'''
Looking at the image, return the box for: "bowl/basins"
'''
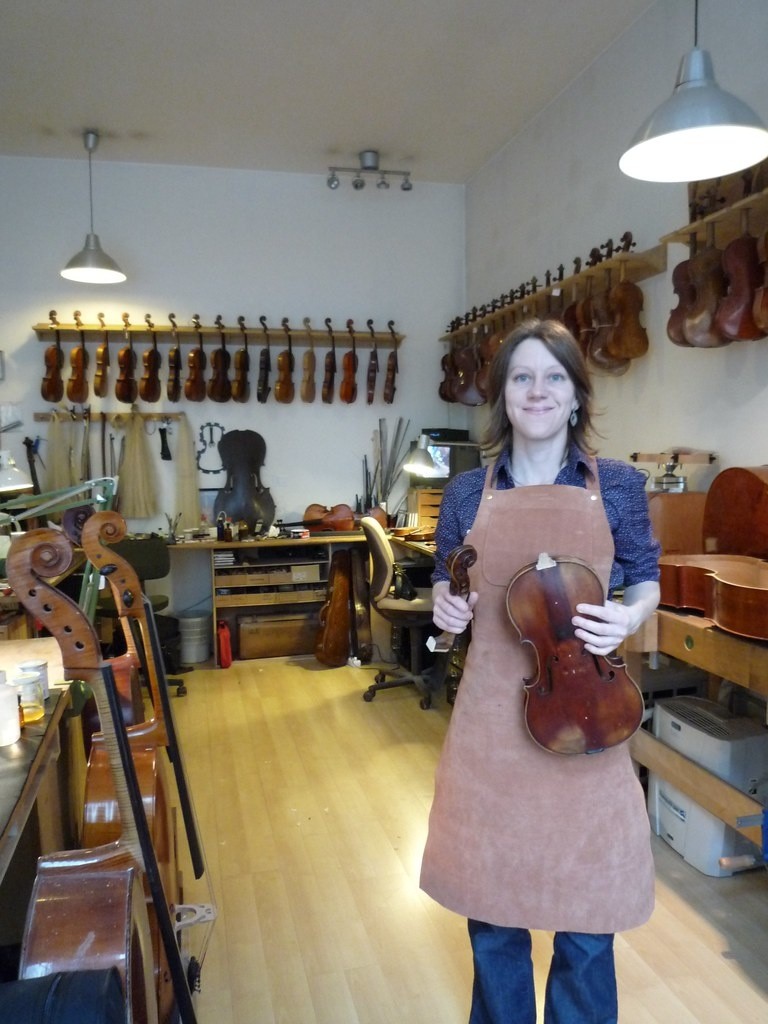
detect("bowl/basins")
[0,582,13,597]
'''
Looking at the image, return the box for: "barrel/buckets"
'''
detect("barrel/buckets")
[176,609,211,663]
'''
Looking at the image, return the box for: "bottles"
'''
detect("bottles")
[20,658,50,699]
[200,514,210,539]
[13,672,45,724]
[0,671,26,747]
[217,516,249,542]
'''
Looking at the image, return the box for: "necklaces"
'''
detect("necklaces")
[508,467,525,485]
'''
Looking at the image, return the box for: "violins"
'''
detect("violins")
[272,502,390,535]
[431,545,648,763]
[394,526,438,541]
[40,311,402,404]
[437,230,647,403]
[667,160,768,350]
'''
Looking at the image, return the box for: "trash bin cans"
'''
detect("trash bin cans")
[175,609,211,664]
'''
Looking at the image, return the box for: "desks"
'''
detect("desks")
[0,638,88,886]
[390,533,436,557]
[615,610,768,861]
[167,535,392,666]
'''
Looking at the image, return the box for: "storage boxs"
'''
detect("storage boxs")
[236,614,321,660]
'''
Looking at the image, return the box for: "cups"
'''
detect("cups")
[183,528,200,541]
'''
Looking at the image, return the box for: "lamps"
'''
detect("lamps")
[619,0,768,183]
[327,151,412,191]
[60,133,127,284]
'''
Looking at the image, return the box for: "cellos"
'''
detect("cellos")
[213,430,276,539]
[702,463,768,555]
[7,516,198,1024]
[656,552,768,643]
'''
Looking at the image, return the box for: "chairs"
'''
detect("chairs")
[361,516,437,710]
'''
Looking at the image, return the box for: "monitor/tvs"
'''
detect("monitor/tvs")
[410,443,481,490]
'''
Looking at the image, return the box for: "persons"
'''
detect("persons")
[430,319,663,1024]
